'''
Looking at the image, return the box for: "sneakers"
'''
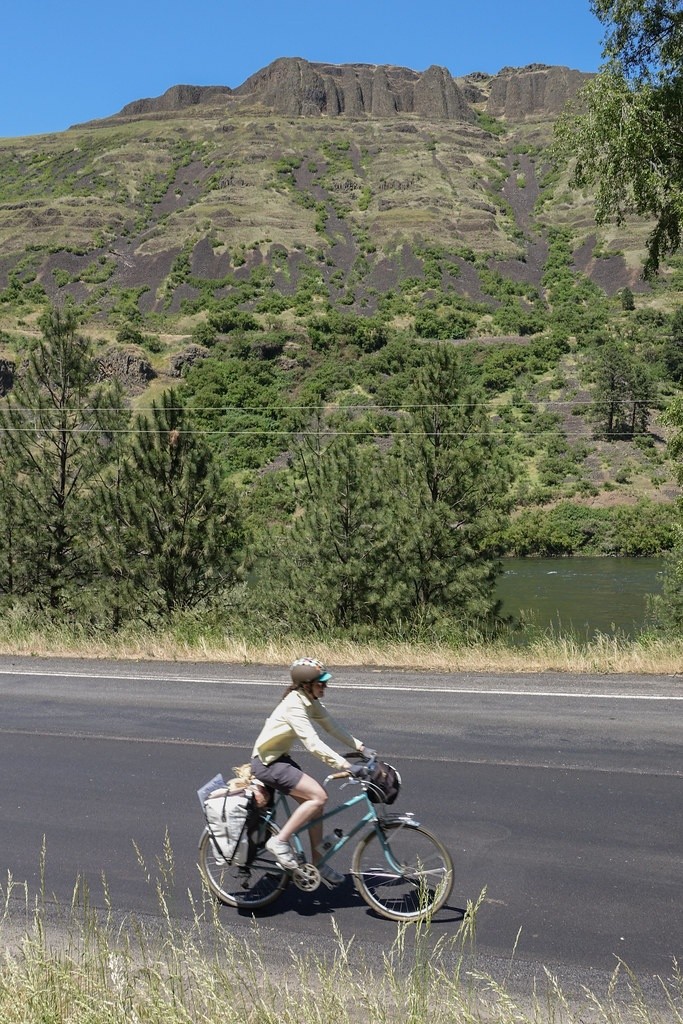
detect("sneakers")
[265,836,299,869]
[320,864,345,883]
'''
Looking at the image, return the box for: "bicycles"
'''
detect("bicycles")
[196,750,455,924]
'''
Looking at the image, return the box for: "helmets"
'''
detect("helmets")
[290,658,332,687]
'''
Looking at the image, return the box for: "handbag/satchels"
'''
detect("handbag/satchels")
[368,761,400,805]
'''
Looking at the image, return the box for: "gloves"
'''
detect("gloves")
[361,747,378,760]
[350,764,365,780]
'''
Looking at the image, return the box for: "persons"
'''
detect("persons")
[250,658,379,883]
